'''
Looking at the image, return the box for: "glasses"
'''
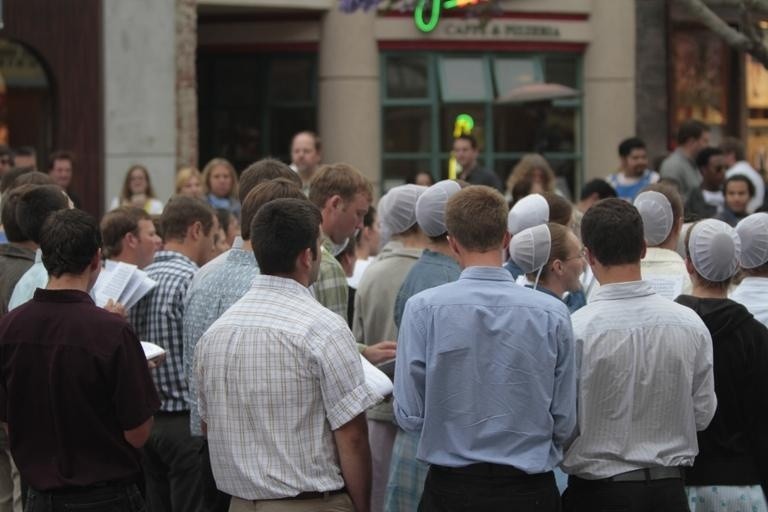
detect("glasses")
[561,247,589,263]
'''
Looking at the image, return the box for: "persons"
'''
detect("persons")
[0,121,767,511]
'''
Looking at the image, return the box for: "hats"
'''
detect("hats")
[634,190,768,281]
[379,180,461,238]
[508,194,551,273]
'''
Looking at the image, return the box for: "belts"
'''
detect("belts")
[265,491,345,499]
[602,466,681,482]
[432,465,527,477]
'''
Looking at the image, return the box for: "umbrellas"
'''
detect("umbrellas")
[495,83,578,105]
